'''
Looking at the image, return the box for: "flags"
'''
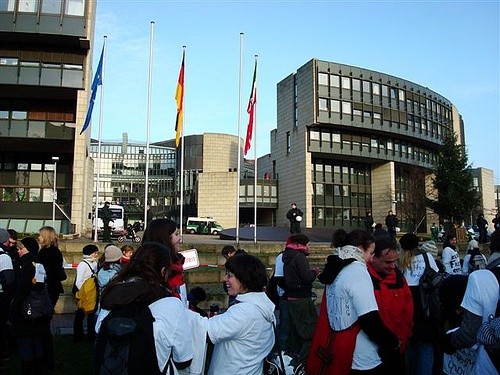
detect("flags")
[79,42,104,136]
[243,59,257,156]
[174,51,184,147]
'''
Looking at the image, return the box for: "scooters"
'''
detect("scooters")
[117,222,142,243]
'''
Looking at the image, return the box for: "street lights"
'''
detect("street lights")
[52,156,59,230]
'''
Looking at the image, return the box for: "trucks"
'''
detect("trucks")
[88,204,128,239]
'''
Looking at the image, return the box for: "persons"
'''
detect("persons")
[0,202,500,375]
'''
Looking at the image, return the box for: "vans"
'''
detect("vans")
[186,216,224,235]
